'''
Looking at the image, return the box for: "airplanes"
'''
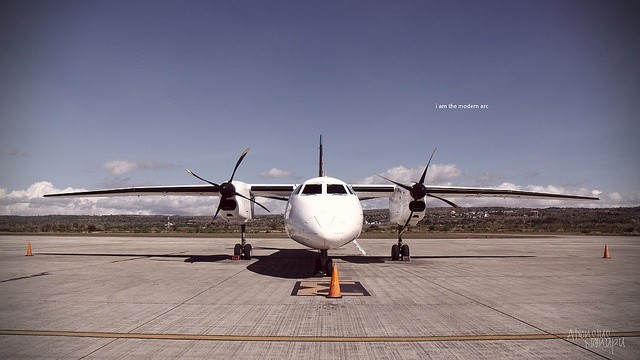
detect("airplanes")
[44,134,599,275]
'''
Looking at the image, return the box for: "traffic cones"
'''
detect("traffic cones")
[326,261,342,299]
[25,241,33,256]
[603,244,610,258]
